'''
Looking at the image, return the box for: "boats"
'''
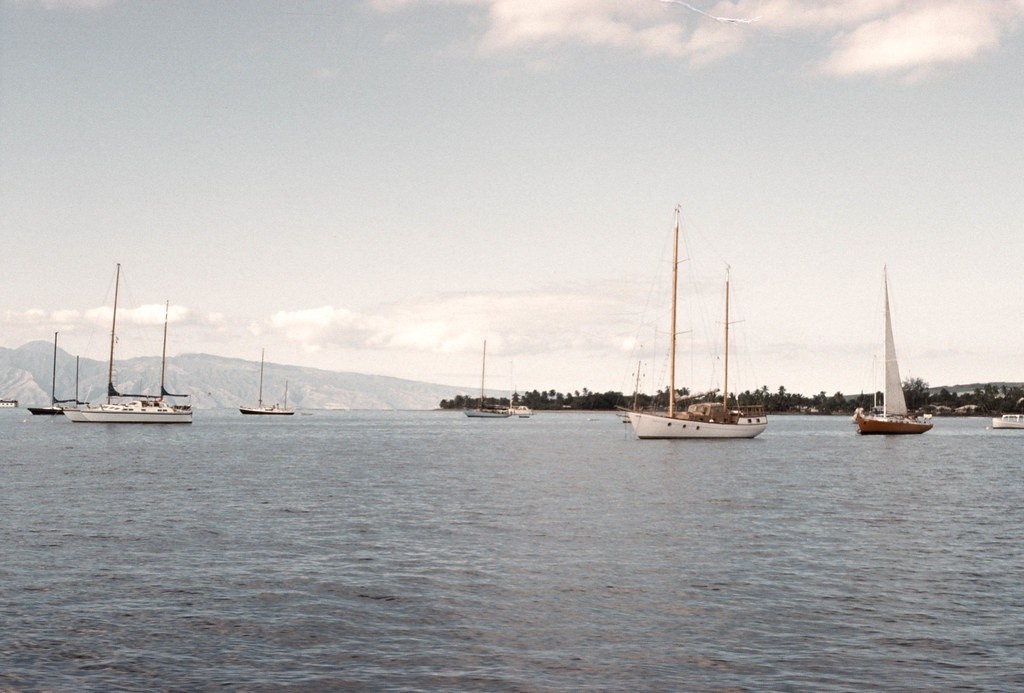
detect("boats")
[495,394,534,415]
[300,412,313,416]
[519,416,530,418]
[992,413,1024,429]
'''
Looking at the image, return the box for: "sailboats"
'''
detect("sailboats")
[868,352,884,418]
[238,347,295,415]
[63,263,195,424]
[26,332,81,415]
[612,202,769,440]
[852,263,934,434]
[462,340,512,418]
